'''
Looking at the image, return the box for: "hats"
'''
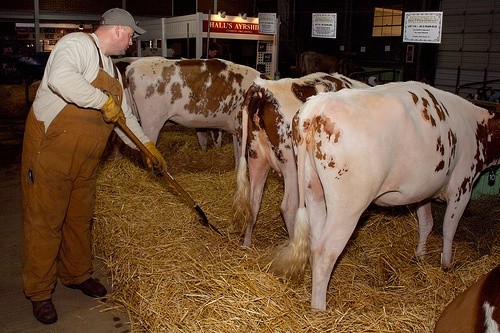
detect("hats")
[100,9,146,35]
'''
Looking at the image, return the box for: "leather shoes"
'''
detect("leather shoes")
[23,291,57,324]
[66,277,107,298]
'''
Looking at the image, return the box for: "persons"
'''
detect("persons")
[23,9,167,324]
[200,42,219,59]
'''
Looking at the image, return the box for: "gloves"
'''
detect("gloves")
[100,94,126,123]
[143,142,168,173]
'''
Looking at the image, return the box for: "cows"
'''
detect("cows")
[230,71,374,247]
[124,57,270,168]
[266,81,500,311]
[434,265,500,333]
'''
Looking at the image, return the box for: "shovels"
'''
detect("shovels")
[117,117,228,238]
